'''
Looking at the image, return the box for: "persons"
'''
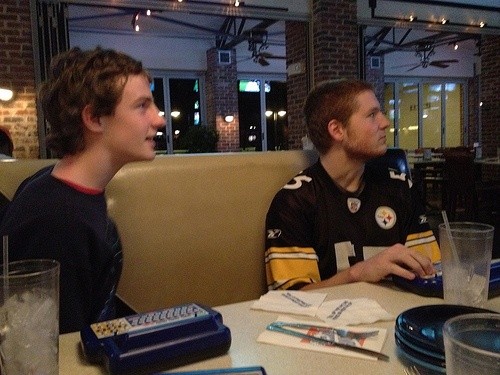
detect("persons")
[264,79,444,290]
[0,45,166,336]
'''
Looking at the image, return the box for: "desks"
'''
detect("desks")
[59,275,500,375]
[474,158,500,226]
[414,158,445,223]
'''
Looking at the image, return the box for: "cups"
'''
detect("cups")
[0,258,59,375]
[438,222,493,309]
[441,313,500,375]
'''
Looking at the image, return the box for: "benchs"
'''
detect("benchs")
[0,149,407,332]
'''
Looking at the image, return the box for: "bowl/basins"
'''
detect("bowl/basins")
[396,304,498,375]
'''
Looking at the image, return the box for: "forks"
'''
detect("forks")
[270,322,379,338]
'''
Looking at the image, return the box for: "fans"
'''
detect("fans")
[390,52,460,72]
[226,43,286,66]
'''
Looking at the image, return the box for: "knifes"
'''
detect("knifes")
[266,325,389,361]
[328,301,352,320]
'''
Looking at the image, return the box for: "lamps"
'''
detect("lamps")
[410,16,417,22]
[448,41,458,50]
[442,19,449,25]
[225,109,234,123]
[479,22,487,28]
[131,9,152,32]
[415,41,435,68]
[248,30,270,64]
[233,1,244,7]
